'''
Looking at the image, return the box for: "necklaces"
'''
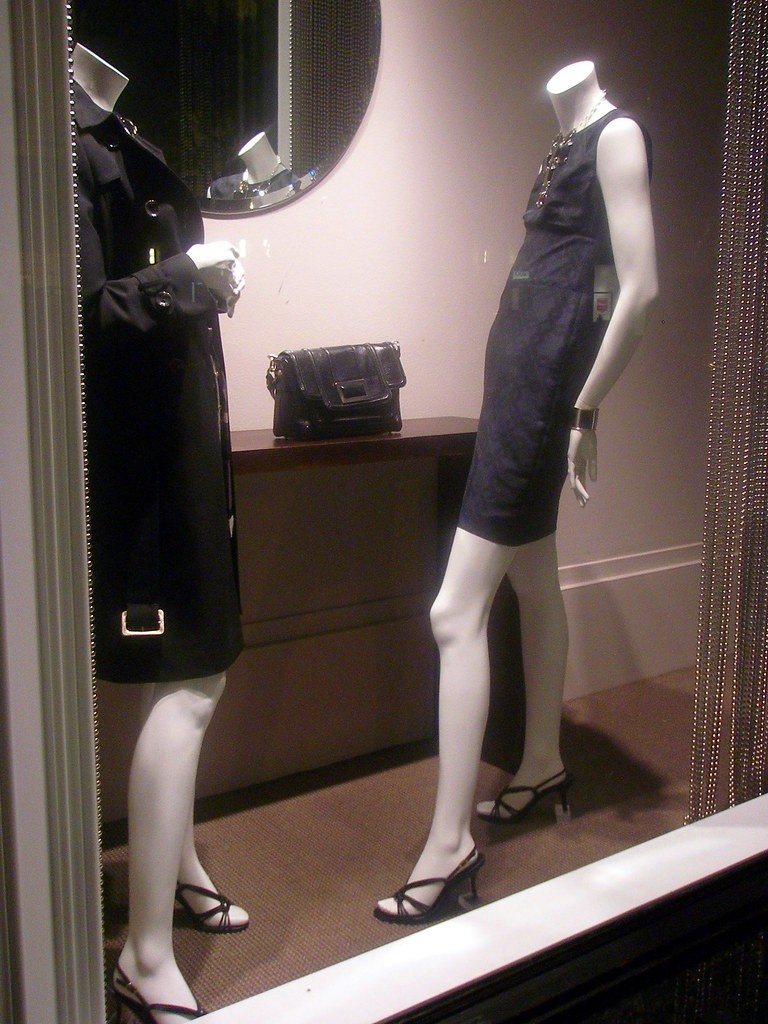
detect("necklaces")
[536,90,607,208]
[240,155,281,199]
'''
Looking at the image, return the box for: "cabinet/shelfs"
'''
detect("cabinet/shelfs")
[93,417,515,840]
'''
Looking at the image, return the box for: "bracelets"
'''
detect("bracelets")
[569,407,599,431]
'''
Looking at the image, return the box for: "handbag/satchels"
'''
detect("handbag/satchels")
[266,340,407,441]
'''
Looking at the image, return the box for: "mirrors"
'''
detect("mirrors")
[68,0,383,218]
[9,0,768,1024]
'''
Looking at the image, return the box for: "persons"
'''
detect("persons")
[71,43,246,1024]
[373,61,659,924]
[207,132,300,199]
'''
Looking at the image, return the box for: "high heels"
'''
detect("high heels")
[175,880,249,932]
[478,767,574,824]
[374,843,486,924]
[112,961,208,1024]
